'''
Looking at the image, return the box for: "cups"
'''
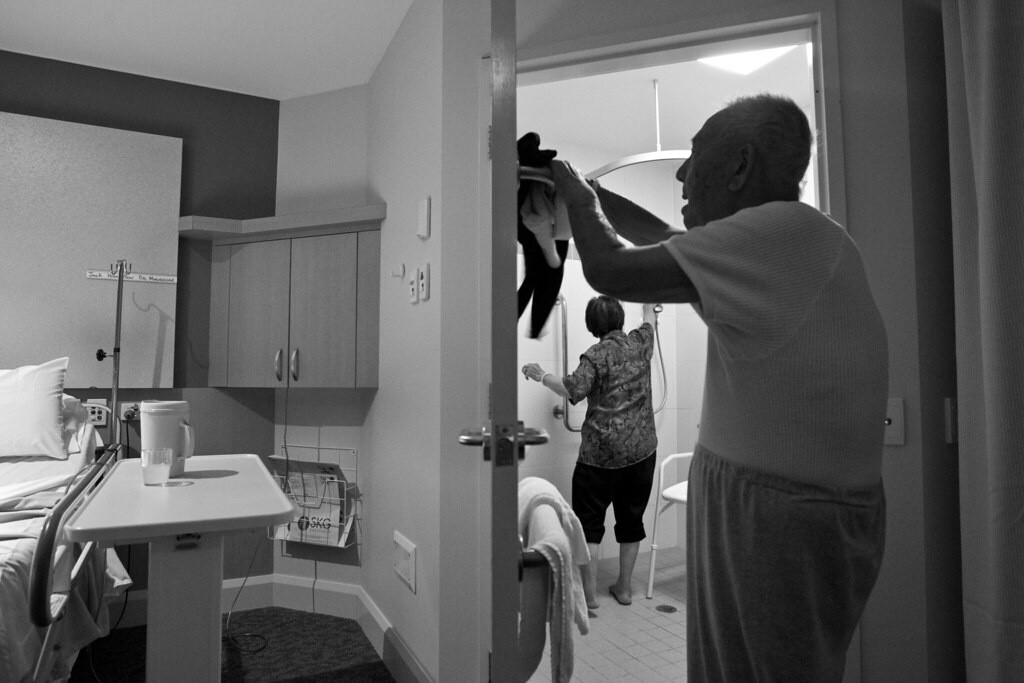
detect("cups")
[141,448,174,488]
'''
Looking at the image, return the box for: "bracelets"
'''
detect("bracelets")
[540,372,550,388]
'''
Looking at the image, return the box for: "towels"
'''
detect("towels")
[516,476,591,683]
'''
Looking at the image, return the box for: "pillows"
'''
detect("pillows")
[0,356,72,459]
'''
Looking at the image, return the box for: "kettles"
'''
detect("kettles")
[139,398,196,477]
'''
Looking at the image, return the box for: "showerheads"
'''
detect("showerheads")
[653,303,664,331]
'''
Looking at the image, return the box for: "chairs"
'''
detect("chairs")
[647,451,696,601]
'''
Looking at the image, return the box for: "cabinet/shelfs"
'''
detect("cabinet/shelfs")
[209,224,379,389]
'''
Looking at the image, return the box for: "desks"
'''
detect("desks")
[63,453,294,683]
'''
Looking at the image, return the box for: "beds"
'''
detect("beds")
[0,403,122,683]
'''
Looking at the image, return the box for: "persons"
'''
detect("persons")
[517,95,889,683]
[521,292,660,610]
